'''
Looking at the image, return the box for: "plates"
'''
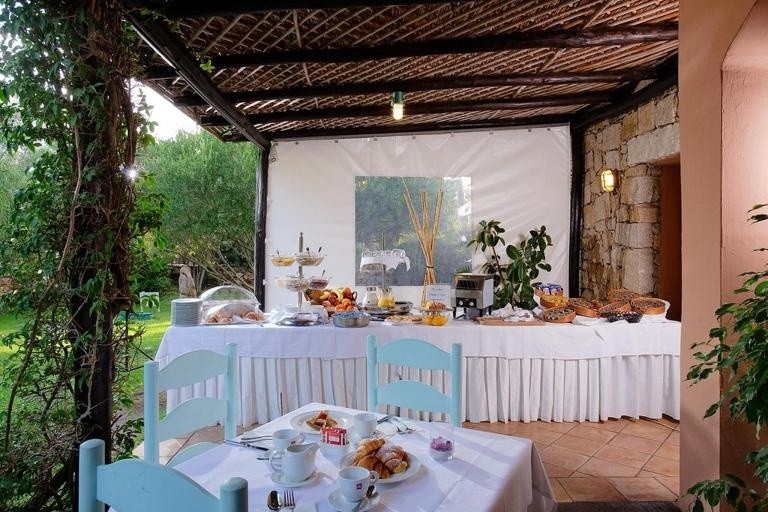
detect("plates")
[339,450,422,483]
[271,470,318,487]
[290,409,354,434]
[327,487,380,511]
[348,421,398,444]
[170,298,203,327]
[200,322,229,325]
[384,318,425,324]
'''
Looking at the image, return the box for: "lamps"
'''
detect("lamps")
[600,168,619,194]
[389,88,406,122]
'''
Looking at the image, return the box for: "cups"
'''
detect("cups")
[269,442,320,481]
[339,466,379,501]
[362,248,406,257]
[272,428,305,448]
[353,413,377,437]
[429,420,454,459]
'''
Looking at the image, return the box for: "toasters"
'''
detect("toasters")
[450,272,493,318]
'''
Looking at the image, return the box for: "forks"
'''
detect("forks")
[283,489,296,512]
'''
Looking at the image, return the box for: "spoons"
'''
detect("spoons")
[352,484,376,511]
[267,490,284,512]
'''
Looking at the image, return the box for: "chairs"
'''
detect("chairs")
[78,439,250,512]
[142,341,239,465]
[364,334,463,427]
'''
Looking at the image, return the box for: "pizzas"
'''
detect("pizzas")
[386,315,422,321]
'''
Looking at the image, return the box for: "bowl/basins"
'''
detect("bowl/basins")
[308,276,332,290]
[284,275,311,292]
[293,253,326,266]
[267,255,296,266]
[332,312,370,328]
[320,439,350,454]
[357,301,414,314]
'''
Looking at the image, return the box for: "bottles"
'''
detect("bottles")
[378,287,395,308]
[361,287,379,308]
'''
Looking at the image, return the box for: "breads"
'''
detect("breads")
[240,310,262,321]
[352,436,409,479]
[208,311,231,323]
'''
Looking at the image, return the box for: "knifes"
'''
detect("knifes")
[223,439,269,451]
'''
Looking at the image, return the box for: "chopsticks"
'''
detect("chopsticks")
[377,414,393,424]
[240,435,272,443]
